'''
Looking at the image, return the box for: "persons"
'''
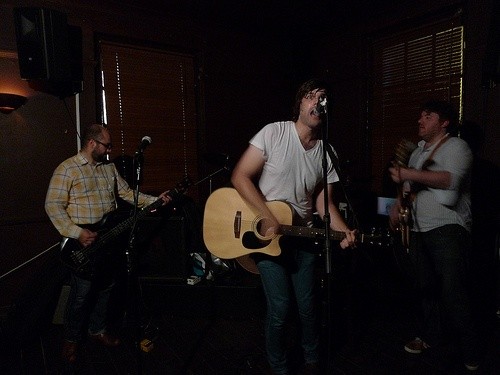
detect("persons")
[45,125,173,366]
[231,78,360,375]
[390,100,482,370]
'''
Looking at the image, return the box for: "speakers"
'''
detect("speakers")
[137,216,189,282]
[13,8,70,81]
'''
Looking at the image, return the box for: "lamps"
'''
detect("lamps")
[0,86,27,111]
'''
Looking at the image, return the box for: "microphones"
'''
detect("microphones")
[314,94,325,117]
[134,136,151,158]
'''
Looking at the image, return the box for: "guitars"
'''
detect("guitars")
[59,175,195,276]
[203,187,395,260]
[395,134,413,257]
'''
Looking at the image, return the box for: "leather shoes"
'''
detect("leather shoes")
[62,341,78,362]
[88,332,120,347]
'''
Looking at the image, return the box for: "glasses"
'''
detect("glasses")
[93,137,112,150]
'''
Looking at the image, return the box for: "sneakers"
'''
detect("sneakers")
[404,337,437,354]
[465,355,481,370]
